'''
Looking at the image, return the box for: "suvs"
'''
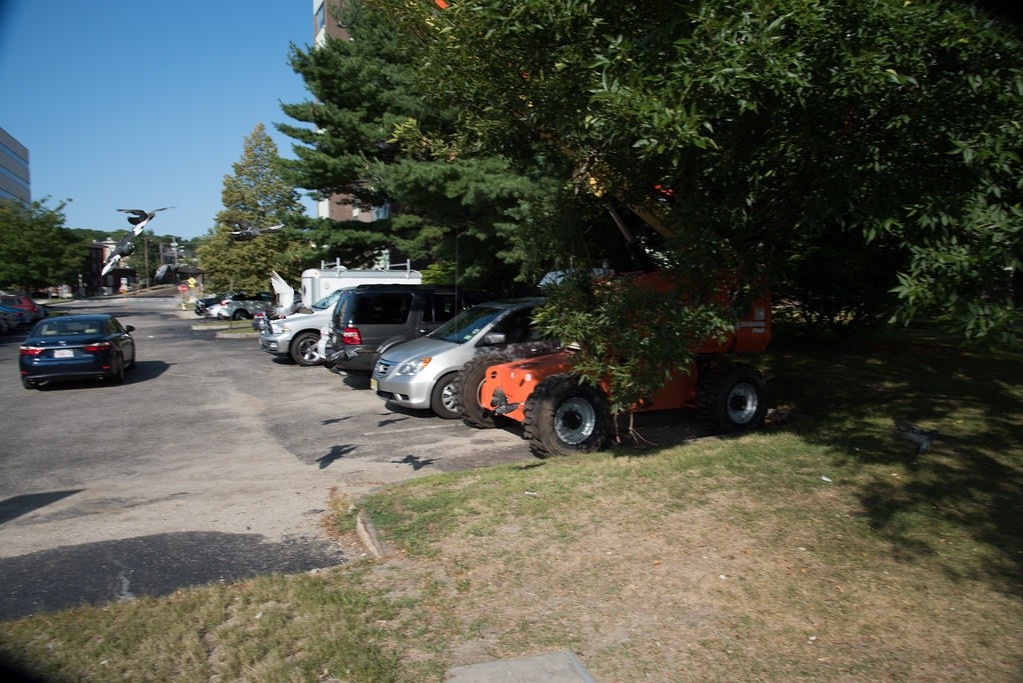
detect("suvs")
[258,287,346,367]
[370,296,569,420]
[324,282,509,375]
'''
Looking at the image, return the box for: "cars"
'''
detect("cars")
[314,323,334,361]
[17,312,136,391]
[0,295,49,335]
[195,292,302,330]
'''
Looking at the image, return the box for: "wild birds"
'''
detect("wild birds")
[153,262,208,281]
[100,218,148,276]
[116,208,167,225]
[217,220,284,243]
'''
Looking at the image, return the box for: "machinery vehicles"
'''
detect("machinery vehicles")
[454,262,780,460]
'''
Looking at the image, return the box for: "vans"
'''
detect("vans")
[299,265,423,315]
